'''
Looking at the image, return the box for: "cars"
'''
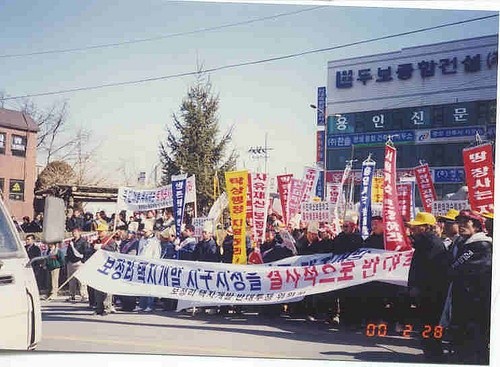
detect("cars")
[1,194,42,351]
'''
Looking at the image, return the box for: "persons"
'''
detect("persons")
[13,209,493,356]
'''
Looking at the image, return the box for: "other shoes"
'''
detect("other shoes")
[40,294,89,304]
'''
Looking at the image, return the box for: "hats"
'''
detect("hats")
[438,208,460,222]
[142,228,153,232]
[405,211,436,226]
[340,221,356,227]
[95,224,108,231]
[160,227,171,239]
[455,209,484,224]
[480,212,493,220]
[317,226,333,235]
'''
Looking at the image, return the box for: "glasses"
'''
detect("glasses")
[202,231,209,235]
[170,235,175,237]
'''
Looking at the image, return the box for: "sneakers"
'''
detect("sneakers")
[88,303,155,317]
